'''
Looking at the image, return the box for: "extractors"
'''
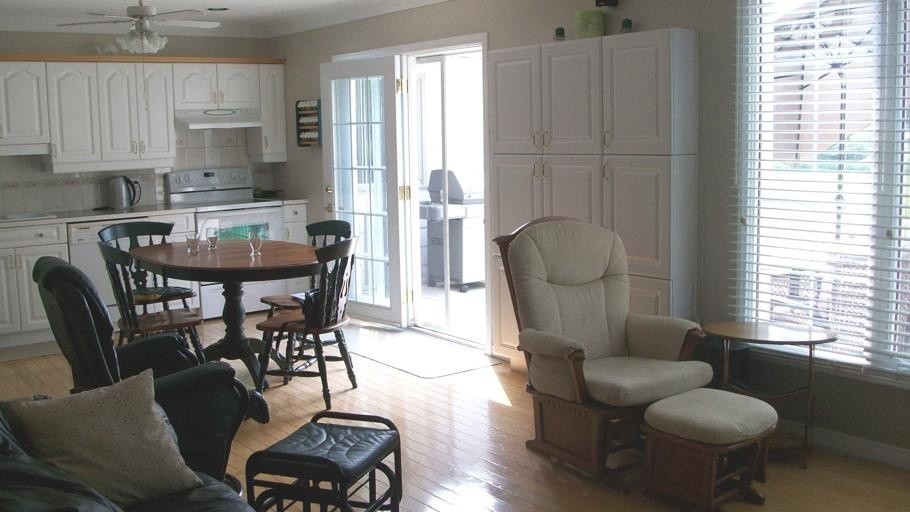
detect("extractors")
[175,108,263,131]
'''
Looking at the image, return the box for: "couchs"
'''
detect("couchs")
[2,363,260,511]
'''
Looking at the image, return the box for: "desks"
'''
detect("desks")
[701,321,842,471]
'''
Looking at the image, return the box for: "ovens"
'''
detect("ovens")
[195,207,290,320]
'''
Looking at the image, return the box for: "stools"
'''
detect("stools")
[243,409,404,512]
[641,386,781,508]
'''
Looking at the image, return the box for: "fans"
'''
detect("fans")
[51,1,222,32]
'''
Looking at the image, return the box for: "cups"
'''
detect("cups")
[248,231,263,257]
[186,232,201,251]
[206,227,219,249]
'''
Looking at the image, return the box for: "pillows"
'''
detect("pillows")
[1,365,205,509]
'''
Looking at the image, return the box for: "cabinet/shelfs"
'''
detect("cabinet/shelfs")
[1,220,70,345]
[487,254,533,367]
[101,62,177,172]
[290,100,322,146]
[627,276,698,316]
[260,63,289,161]
[488,151,601,258]
[1,58,49,154]
[603,153,699,279]
[172,62,261,110]
[286,199,311,295]
[46,62,100,173]
[489,36,602,154]
[602,31,699,156]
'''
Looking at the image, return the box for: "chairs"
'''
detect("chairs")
[258,217,354,370]
[96,219,199,351]
[96,239,205,360]
[31,250,268,495]
[491,212,713,493]
[251,235,361,410]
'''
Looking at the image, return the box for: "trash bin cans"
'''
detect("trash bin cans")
[427,169,486,293]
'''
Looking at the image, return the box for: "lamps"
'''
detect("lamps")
[116,19,169,55]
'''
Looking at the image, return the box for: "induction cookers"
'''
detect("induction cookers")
[166,189,283,212]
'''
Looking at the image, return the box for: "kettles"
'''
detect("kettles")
[105,175,136,211]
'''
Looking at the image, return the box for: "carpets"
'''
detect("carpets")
[305,326,504,381]
